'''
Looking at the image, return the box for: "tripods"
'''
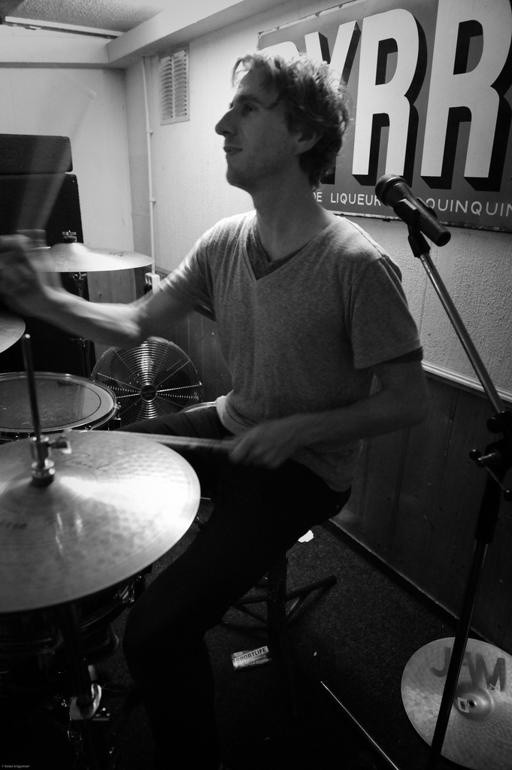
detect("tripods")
[206,539,336,712]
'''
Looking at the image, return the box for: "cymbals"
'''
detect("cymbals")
[401,637,512,770]
[28,242,153,274]
[0,429,201,614]
[0,312,26,351]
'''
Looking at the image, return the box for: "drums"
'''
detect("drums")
[0,370,121,445]
[0,565,151,656]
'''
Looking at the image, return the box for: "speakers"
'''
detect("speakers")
[0,173,97,377]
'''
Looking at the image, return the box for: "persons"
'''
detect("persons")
[3,51,436,769]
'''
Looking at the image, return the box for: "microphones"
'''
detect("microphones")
[374,174,451,247]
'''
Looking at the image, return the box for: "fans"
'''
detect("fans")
[87,335,205,429]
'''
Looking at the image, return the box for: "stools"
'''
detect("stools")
[217,484,356,722]
